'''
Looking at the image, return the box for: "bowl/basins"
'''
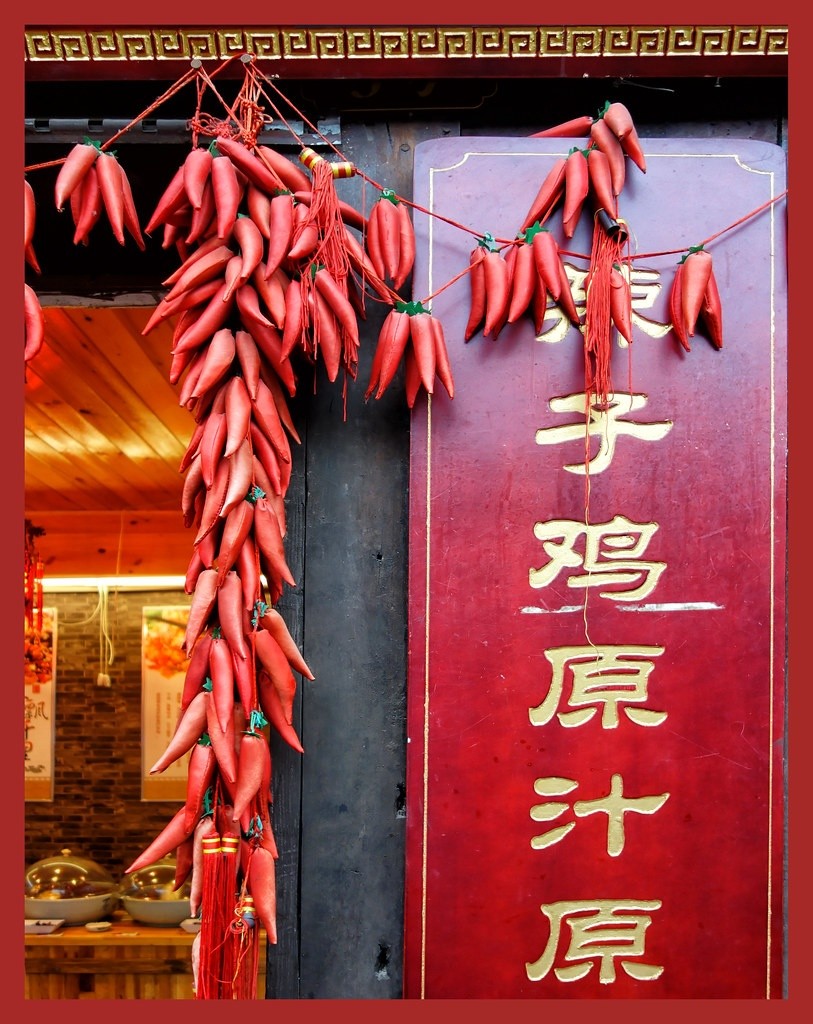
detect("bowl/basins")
[116,882,202,926]
[25,880,125,926]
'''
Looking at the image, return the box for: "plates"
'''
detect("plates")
[24,919,63,934]
[84,921,111,930]
[179,917,203,933]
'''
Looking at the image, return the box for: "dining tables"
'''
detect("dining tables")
[24,910,266,1001]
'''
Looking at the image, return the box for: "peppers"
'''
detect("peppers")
[24,102,725,972]
